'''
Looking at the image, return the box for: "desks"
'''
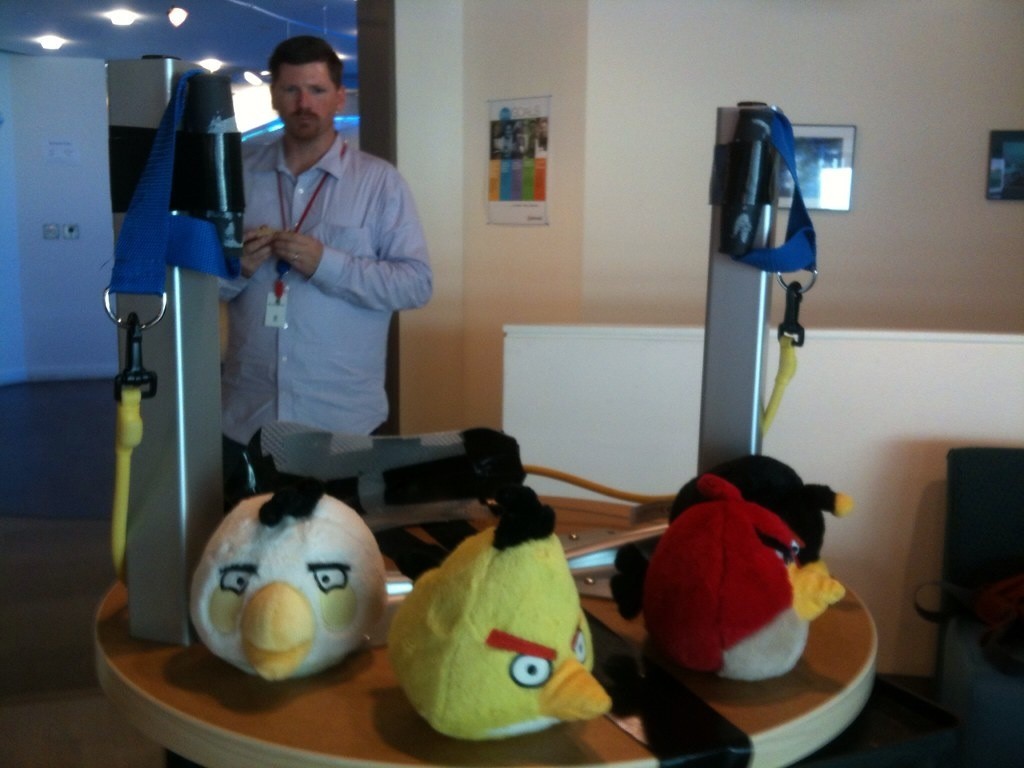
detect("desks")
[87,495,880,767]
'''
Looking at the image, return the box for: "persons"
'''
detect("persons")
[490,117,548,158]
[217,33,432,495]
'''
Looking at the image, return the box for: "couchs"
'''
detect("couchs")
[935,445,1024,768]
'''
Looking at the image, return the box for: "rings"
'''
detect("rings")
[294,252,299,259]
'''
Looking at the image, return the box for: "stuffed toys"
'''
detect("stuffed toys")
[190,479,385,683]
[639,472,807,678]
[666,454,852,622]
[388,484,613,741]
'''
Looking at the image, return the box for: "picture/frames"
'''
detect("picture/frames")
[777,123,855,211]
[986,129,1024,200]
